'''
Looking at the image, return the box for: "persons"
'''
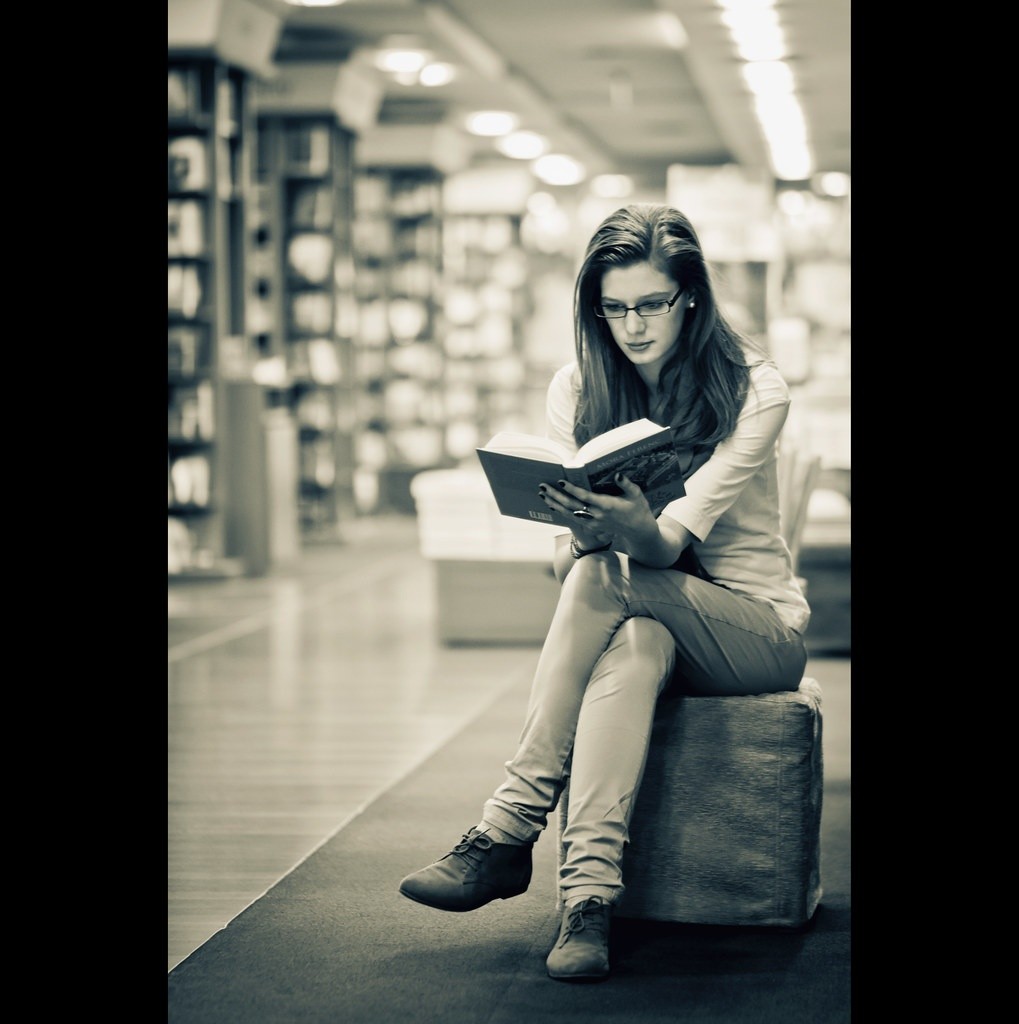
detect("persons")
[401,202,811,981]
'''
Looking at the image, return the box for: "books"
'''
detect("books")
[169,63,541,577]
[477,419,688,530]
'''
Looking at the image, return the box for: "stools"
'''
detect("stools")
[556,678,826,929]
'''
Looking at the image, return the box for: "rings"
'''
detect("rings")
[573,502,595,520]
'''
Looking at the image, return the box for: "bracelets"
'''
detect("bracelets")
[571,536,614,559]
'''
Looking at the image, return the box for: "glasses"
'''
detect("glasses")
[592,288,685,319]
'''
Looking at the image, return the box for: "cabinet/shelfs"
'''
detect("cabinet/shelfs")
[168,43,259,582]
[256,107,361,534]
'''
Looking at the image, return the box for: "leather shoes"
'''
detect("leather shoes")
[546,894,609,978]
[398,825,534,912]
